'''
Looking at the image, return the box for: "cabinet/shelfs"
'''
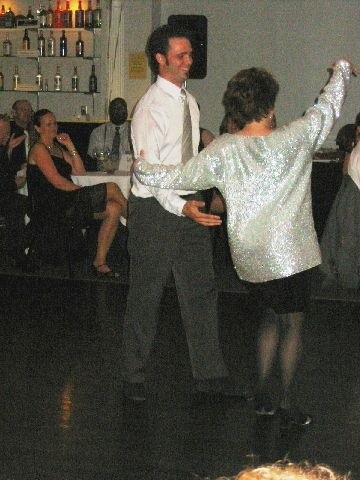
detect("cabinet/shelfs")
[0,26,102,115]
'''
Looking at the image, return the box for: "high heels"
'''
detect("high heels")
[91,264,120,278]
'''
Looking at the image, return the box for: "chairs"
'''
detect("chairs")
[22,168,91,279]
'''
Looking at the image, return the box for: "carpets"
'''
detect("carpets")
[0,222,360,302]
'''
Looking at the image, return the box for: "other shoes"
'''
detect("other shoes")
[275,407,312,426]
[245,395,275,415]
[197,380,255,397]
[122,382,147,401]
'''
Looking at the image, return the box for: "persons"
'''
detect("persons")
[26,109,128,278]
[336,112,360,190]
[199,114,244,214]
[7,99,33,173]
[133,55,360,430]
[86,97,132,163]
[0,114,40,274]
[118,26,247,408]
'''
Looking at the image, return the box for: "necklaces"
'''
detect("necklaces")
[45,144,60,152]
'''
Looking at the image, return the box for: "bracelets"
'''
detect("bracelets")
[70,149,78,156]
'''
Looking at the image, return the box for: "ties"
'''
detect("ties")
[24,129,30,162]
[110,127,120,171]
[182,89,193,166]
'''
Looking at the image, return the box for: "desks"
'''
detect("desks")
[16,170,132,254]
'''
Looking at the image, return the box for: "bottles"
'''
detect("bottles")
[37,29,45,56]
[89,64,98,92]
[76,31,85,56]
[35,62,43,91]
[3,31,13,56]
[0,0,101,28]
[13,64,20,90]
[23,28,30,51]
[48,30,57,56]
[71,63,80,92]
[0,71,3,91]
[54,65,62,92]
[59,30,67,57]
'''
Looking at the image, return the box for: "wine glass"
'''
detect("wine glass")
[94,144,110,173]
